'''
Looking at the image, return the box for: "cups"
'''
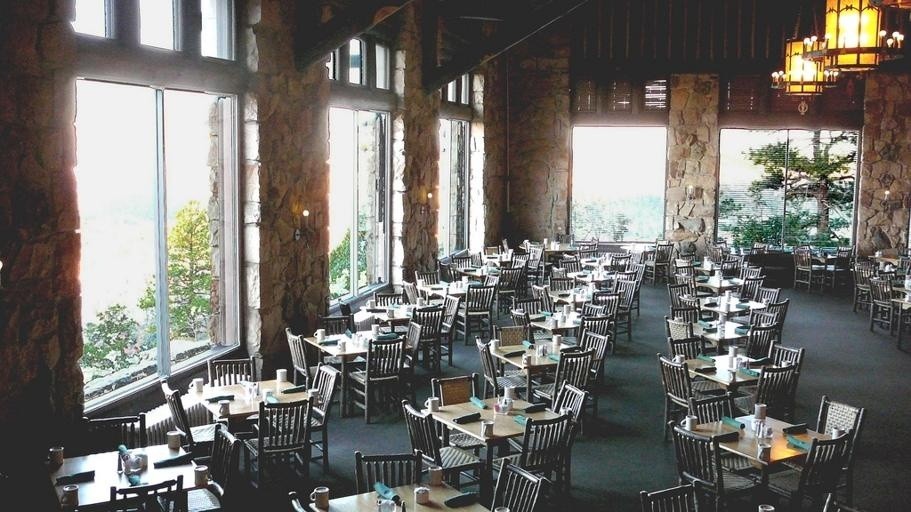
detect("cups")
[307,462,512,512]
[671,342,792,380]
[363,296,447,318]
[512,304,608,328]
[678,402,848,461]
[489,332,584,368]
[44,428,211,507]
[672,314,774,339]
[757,504,775,511]
[311,322,406,353]
[188,367,323,416]
[424,383,573,437]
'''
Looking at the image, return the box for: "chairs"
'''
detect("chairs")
[40,232,911,512]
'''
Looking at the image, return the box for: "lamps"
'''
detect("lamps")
[795,0,911,82]
[767,3,843,118]
